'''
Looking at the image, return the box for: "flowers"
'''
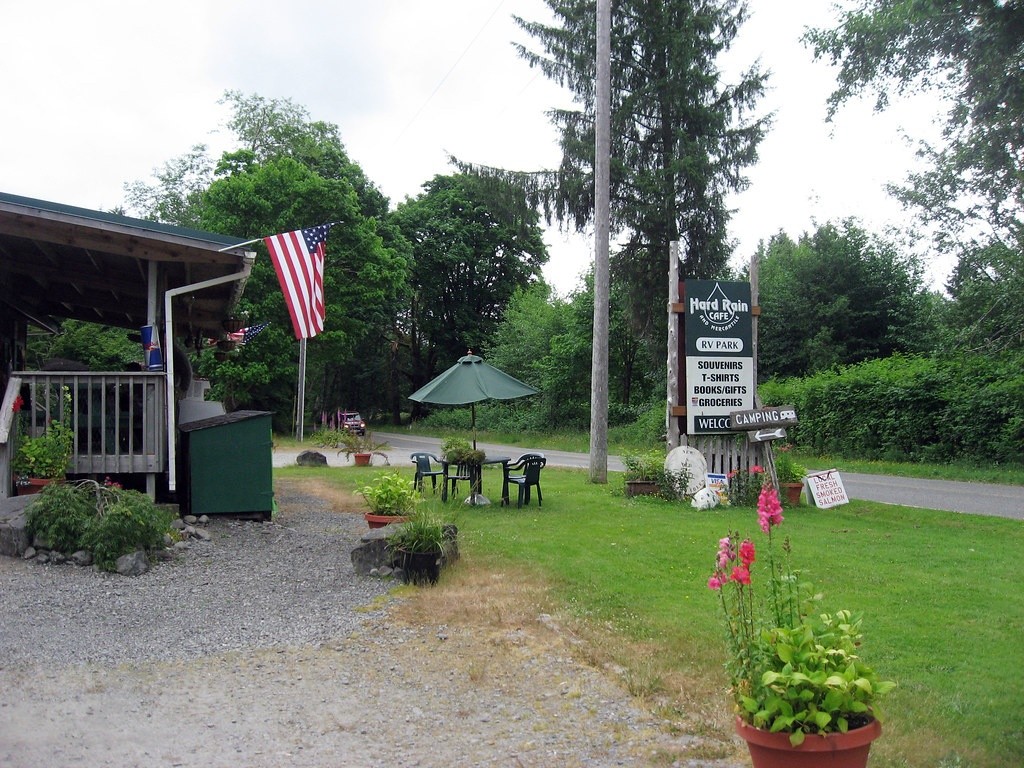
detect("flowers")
[11,386,75,486]
[708,483,897,748]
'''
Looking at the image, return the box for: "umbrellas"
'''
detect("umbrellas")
[408,349,540,451]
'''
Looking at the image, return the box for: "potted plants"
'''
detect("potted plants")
[779,463,808,506]
[337,428,390,466]
[353,469,426,529]
[383,503,463,585]
[619,445,664,495]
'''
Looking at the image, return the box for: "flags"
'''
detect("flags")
[265,222,333,341]
[207,323,268,346]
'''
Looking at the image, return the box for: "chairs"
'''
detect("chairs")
[410,453,444,494]
[501,452,546,509]
[447,463,471,499]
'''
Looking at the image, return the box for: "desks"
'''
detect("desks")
[441,457,511,507]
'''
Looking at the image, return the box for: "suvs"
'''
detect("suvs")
[334,411,367,436]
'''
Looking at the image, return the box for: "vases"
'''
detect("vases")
[735,713,881,768]
[13,473,66,495]
